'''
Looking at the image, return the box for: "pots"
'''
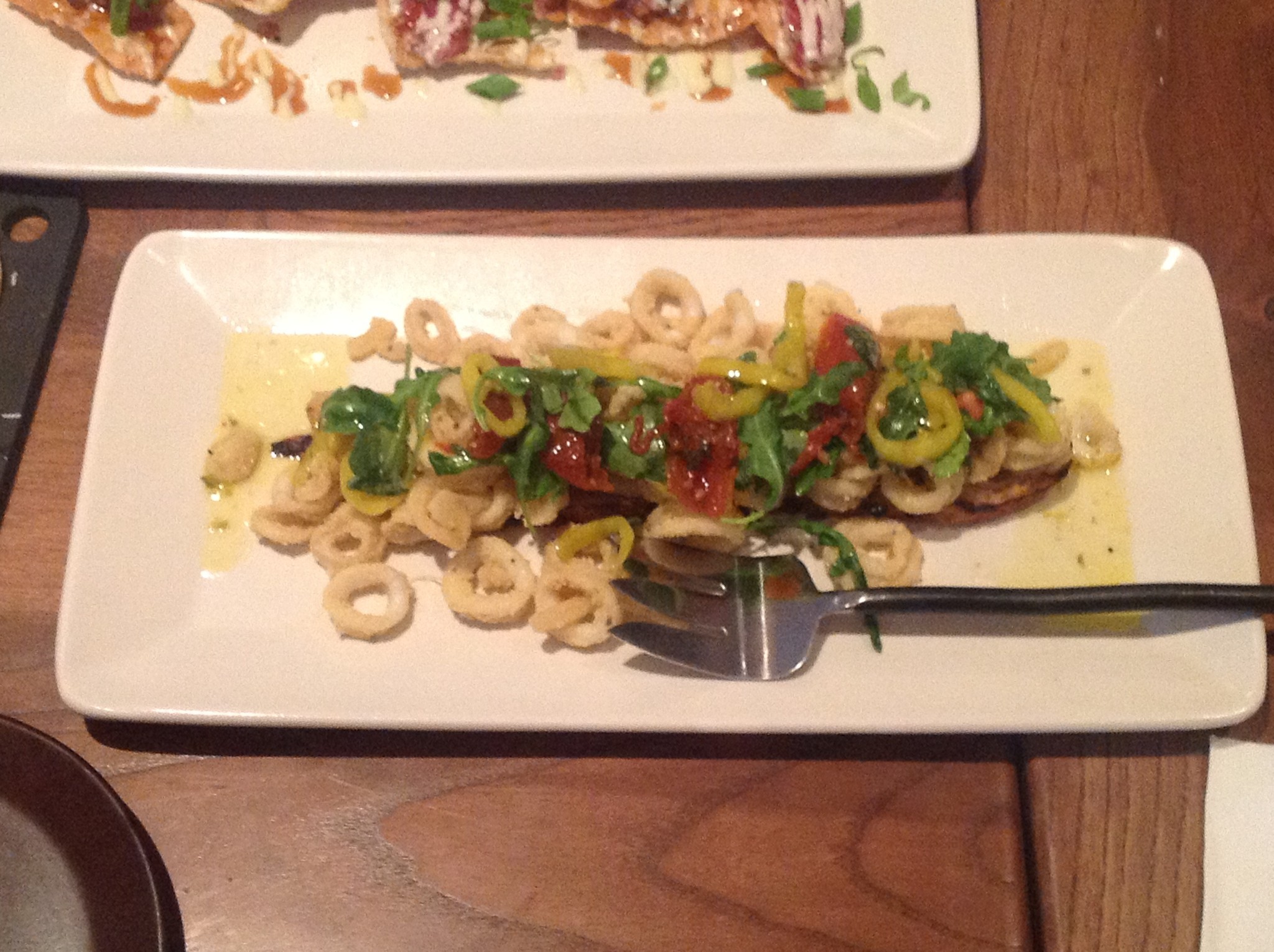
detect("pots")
[0,185,188,952]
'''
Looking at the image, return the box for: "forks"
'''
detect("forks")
[607,539,1274,680]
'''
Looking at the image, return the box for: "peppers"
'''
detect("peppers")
[340,280,1053,584]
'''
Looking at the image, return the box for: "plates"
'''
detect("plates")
[0,0,986,183]
[58,226,1271,737]
